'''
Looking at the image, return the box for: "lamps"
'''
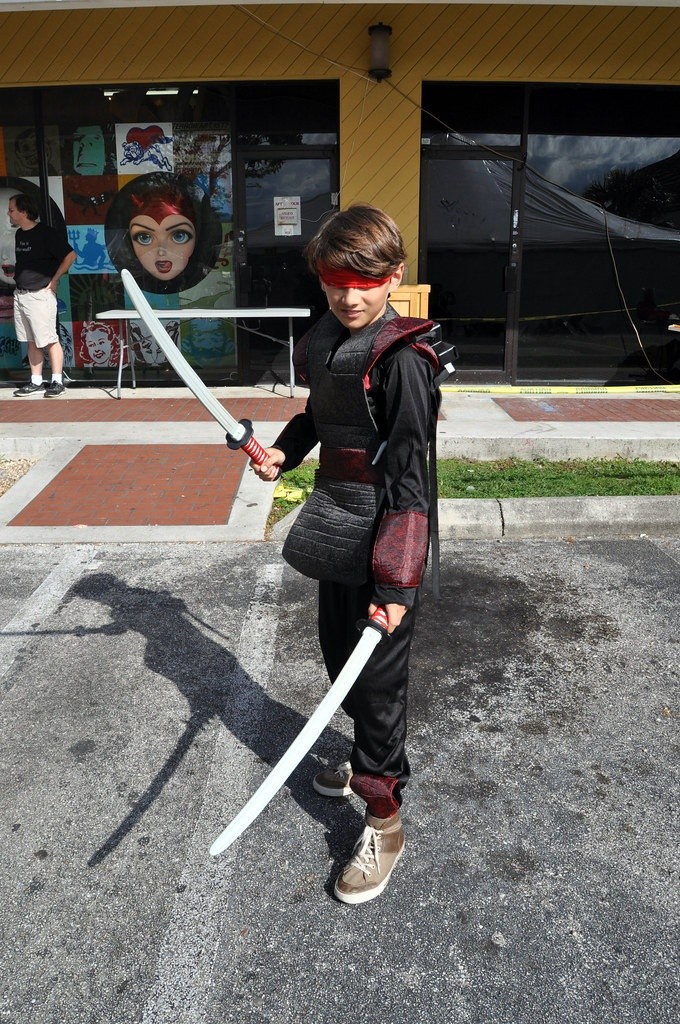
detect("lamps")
[367,21,392,84]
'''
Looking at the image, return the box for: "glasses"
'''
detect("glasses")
[8,207,20,213]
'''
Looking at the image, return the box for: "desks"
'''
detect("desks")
[95,308,310,399]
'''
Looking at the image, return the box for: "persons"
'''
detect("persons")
[5,193,79,400]
[247,203,457,909]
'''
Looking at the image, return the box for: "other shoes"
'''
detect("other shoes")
[44,381,67,399]
[334,810,409,903]
[14,383,48,397]
[314,759,355,797]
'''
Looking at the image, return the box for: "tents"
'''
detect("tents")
[243,145,680,254]
